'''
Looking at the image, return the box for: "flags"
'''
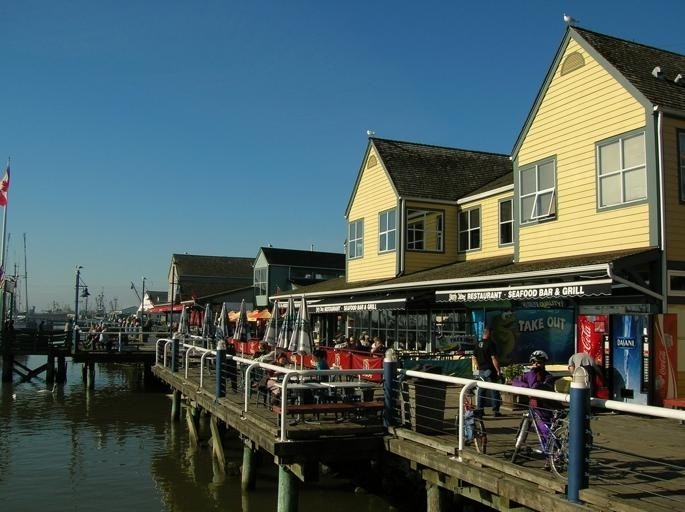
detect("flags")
[0,160,11,206]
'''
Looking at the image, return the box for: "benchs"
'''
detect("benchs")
[272,401,401,427]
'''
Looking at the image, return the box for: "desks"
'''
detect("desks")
[269,375,380,421]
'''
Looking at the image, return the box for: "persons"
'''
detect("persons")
[62,315,155,352]
[472,328,506,417]
[370,336,385,349]
[371,339,387,353]
[568,352,600,416]
[215,339,238,392]
[348,336,364,350]
[236,340,268,389]
[358,335,371,350]
[289,349,329,406]
[510,349,565,470]
[265,352,289,411]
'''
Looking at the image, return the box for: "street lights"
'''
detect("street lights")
[69,264,91,326]
[128,276,147,328]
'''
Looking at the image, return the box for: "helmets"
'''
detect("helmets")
[529,350,549,362]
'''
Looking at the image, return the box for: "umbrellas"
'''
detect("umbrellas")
[177,304,190,348]
[261,299,282,360]
[201,303,216,355]
[275,294,298,370]
[187,304,203,356]
[217,301,231,349]
[232,298,252,359]
[288,294,315,370]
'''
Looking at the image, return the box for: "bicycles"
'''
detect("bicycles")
[454,385,489,457]
[509,395,570,485]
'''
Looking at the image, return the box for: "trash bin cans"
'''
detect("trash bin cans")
[394,365,447,435]
